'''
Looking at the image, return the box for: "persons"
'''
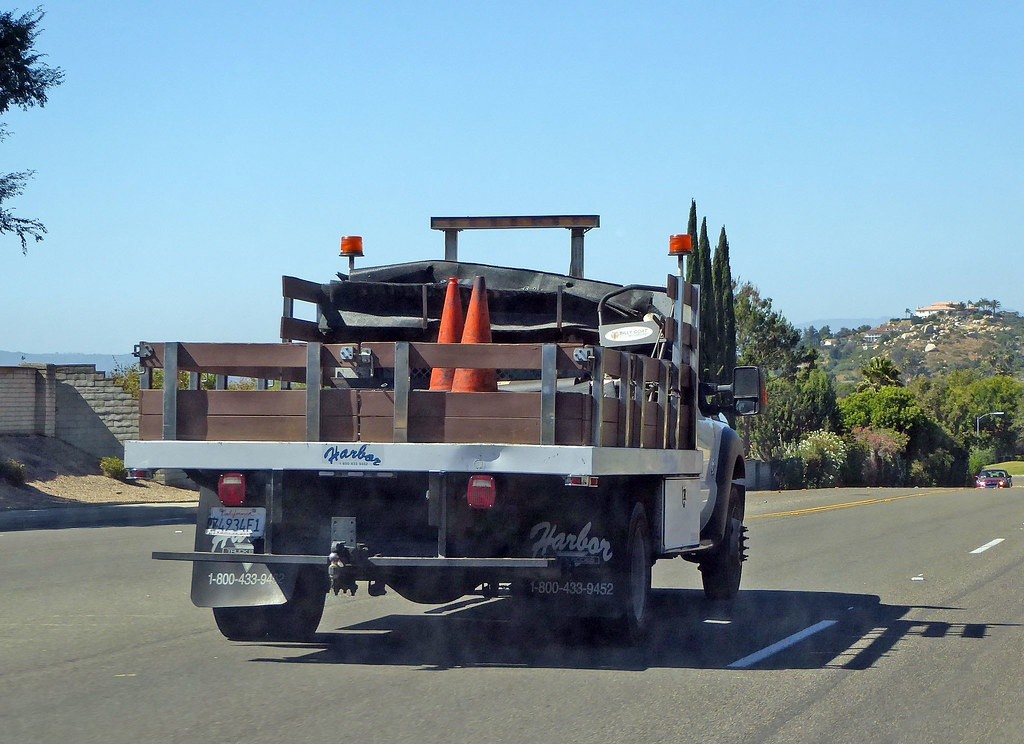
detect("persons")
[575,312,660,385]
[998,473,1004,478]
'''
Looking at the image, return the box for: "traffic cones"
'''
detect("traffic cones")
[452,275,498,393]
[429,278,464,391]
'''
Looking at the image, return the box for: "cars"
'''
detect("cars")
[974,469,1013,488]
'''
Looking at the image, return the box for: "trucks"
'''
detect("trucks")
[124,215,772,642]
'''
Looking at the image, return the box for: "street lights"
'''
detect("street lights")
[977,412,1004,435]
